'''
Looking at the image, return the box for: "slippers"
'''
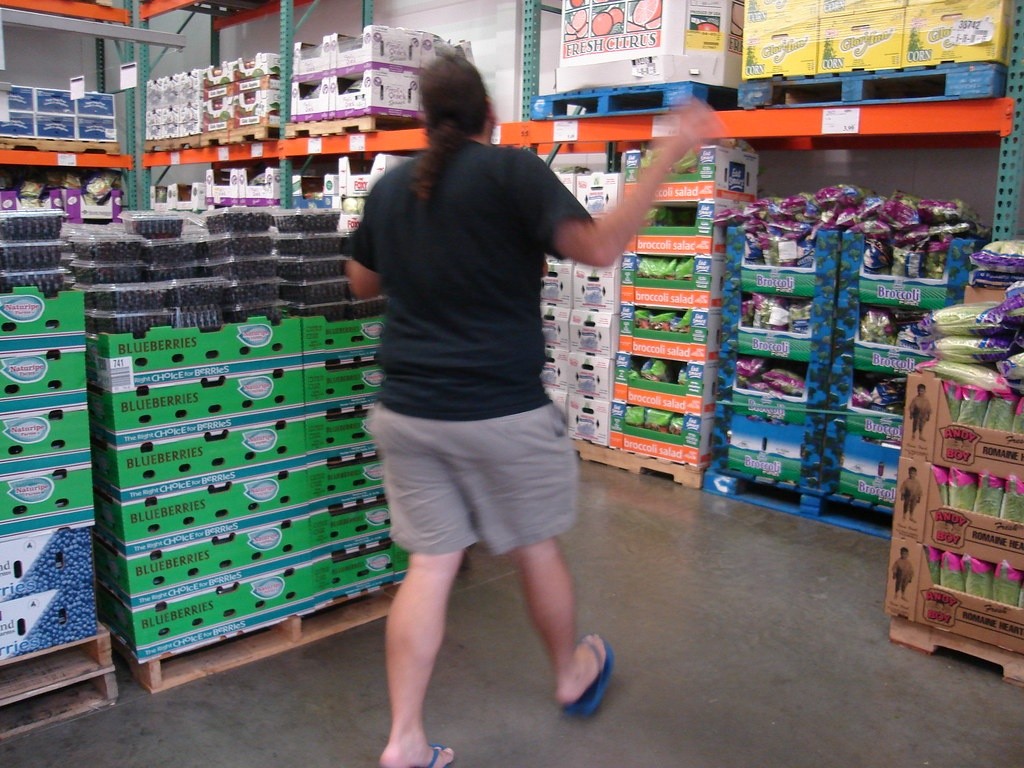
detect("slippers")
[426,744,448,768]
[562,636,612,719]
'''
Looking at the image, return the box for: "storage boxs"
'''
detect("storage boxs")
[0,0,1024,744]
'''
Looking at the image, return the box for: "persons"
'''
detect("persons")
[340,54,715,768]
